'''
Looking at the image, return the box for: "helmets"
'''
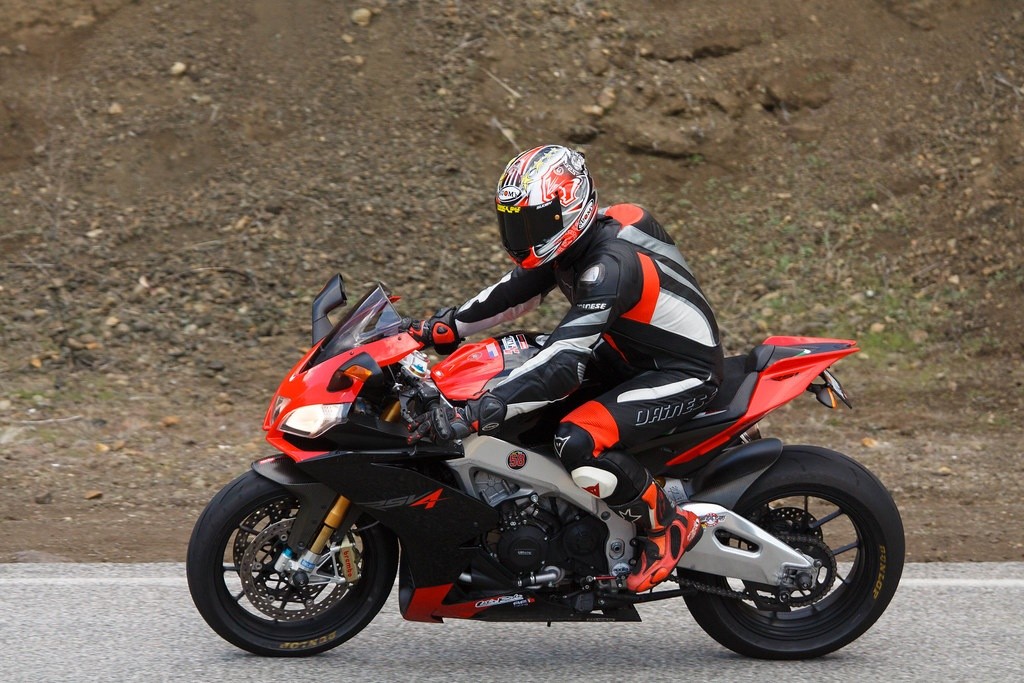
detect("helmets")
[493,144,600,270]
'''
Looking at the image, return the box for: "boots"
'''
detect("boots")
[607,466,704,592]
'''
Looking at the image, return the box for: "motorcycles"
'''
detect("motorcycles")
[186,271,909,663]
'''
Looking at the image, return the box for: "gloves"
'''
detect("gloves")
[398,305,461,355]
[406,388,508,448]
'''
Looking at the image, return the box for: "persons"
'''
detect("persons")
[375,144,725,592]
